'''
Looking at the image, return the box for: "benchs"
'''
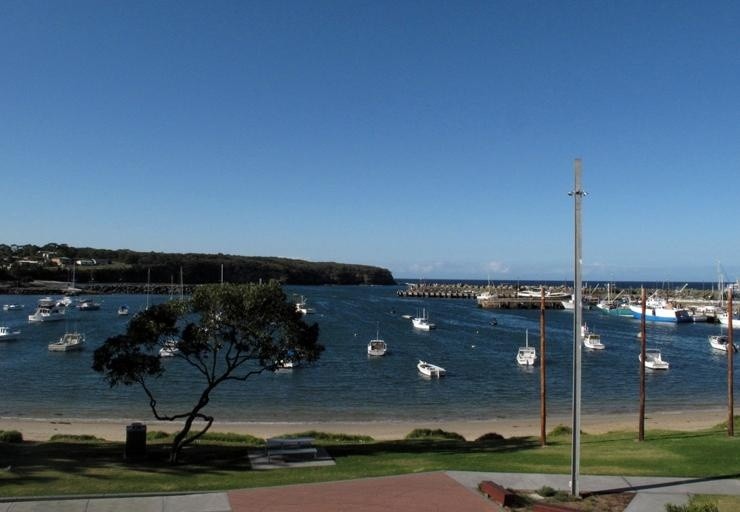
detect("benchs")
[480,478,513,507]
[267,448,318,463]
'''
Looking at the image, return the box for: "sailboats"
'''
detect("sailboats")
[597,284,740,330]
[412,306,436,330]
[515,326,535,365]
[60,262,83,294]
[367,320,386,356]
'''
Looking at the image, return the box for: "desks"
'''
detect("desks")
[268,435,315,448]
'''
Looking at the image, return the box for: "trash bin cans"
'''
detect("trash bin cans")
[125,422,146,462]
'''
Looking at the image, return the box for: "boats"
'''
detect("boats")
[638,348,669,370]
[0,326,21,341]
[707,334,738,351]
[29,297,62,322]
[561,294,590,310]
[118,303,129,315]
[418,358,447,378]
[3,304,22,311]
[159,339,178,358]
[48,331,85,353]
[294,301,313,315]
[77,299,100,311]
[274,349,299,368]
[57,294,71,308]
[582,324,605,350]
